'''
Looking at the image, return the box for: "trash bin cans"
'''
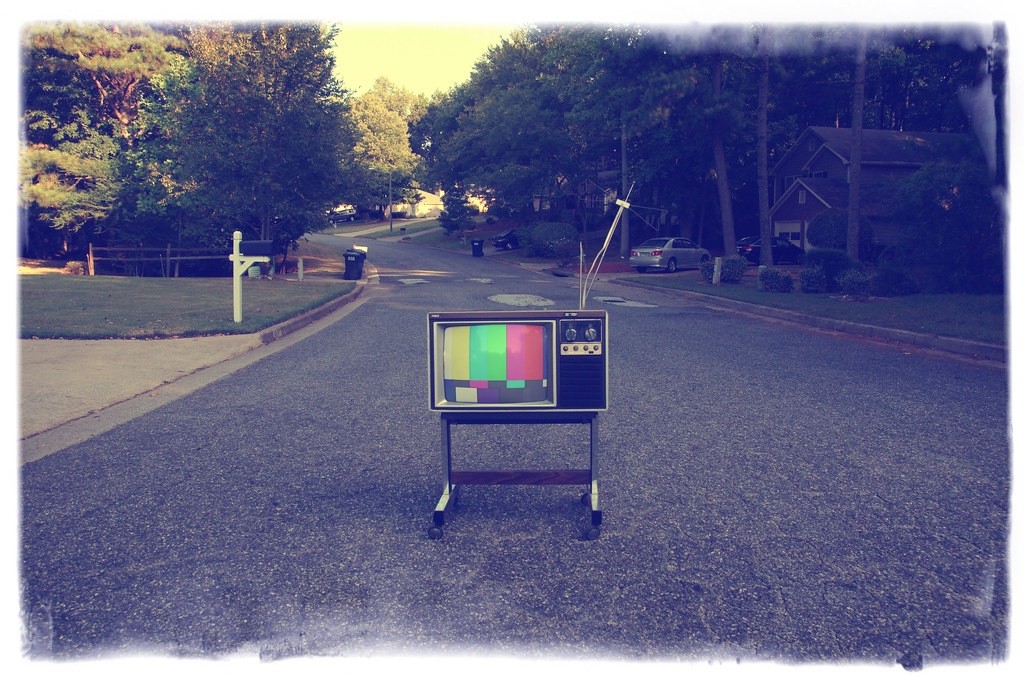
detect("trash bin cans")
[471,239,484,257]
[343,248,367,280]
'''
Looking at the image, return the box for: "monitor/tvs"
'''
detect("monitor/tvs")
[427,178,668,412]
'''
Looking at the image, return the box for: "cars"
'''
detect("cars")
[492,227,519,249]
[736,236,805,264]
[327,209,357,223]
[630,236,712,273]
